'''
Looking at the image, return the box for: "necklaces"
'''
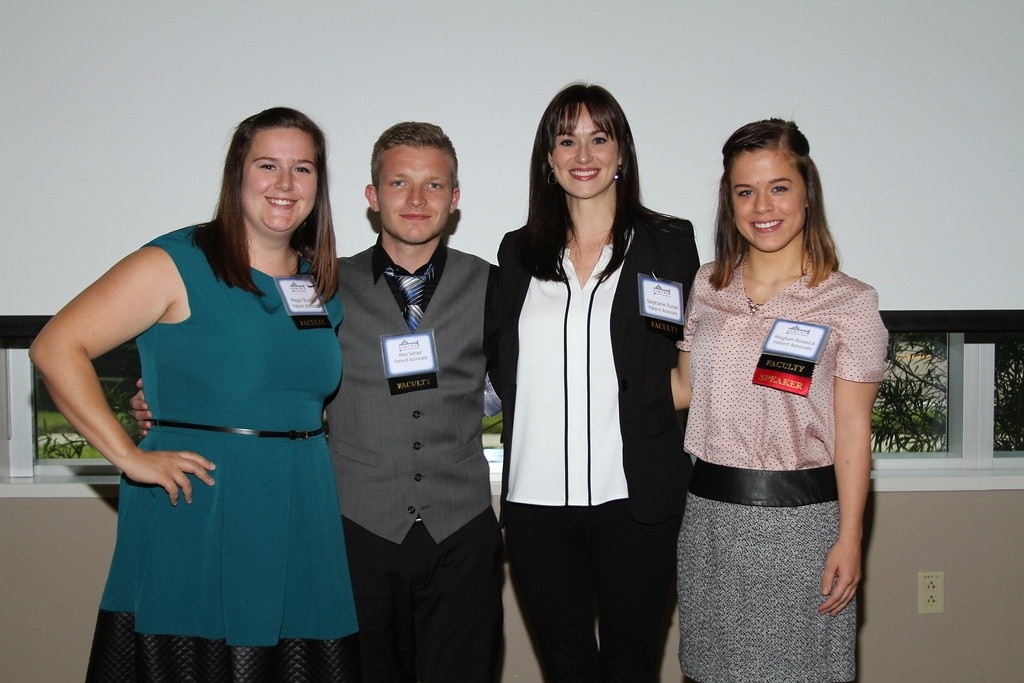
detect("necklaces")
[747,298,762,310]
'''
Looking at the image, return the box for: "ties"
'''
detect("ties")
[382,261,435,334]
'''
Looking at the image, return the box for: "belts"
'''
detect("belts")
[148,419,324,441]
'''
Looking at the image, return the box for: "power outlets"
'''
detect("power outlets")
[916,571,944,615]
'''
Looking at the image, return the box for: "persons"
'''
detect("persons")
[132,122,504,683]
[671,118,888,683]
[28,107,364,683]
[494,85,702,683]
